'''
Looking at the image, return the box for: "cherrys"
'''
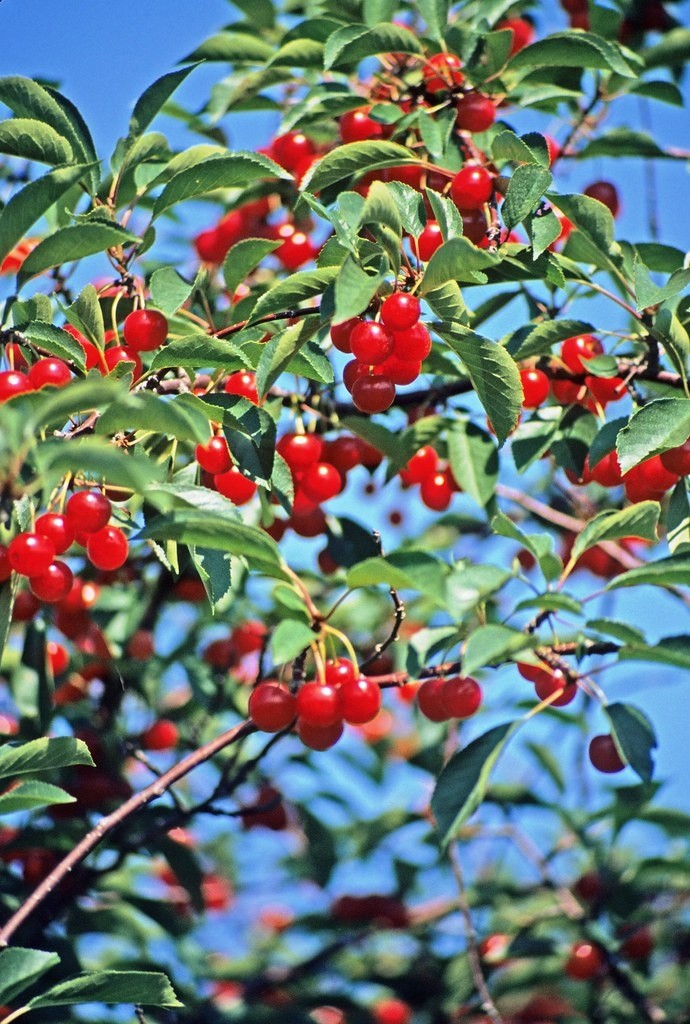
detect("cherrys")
[0,0,690,1024]
